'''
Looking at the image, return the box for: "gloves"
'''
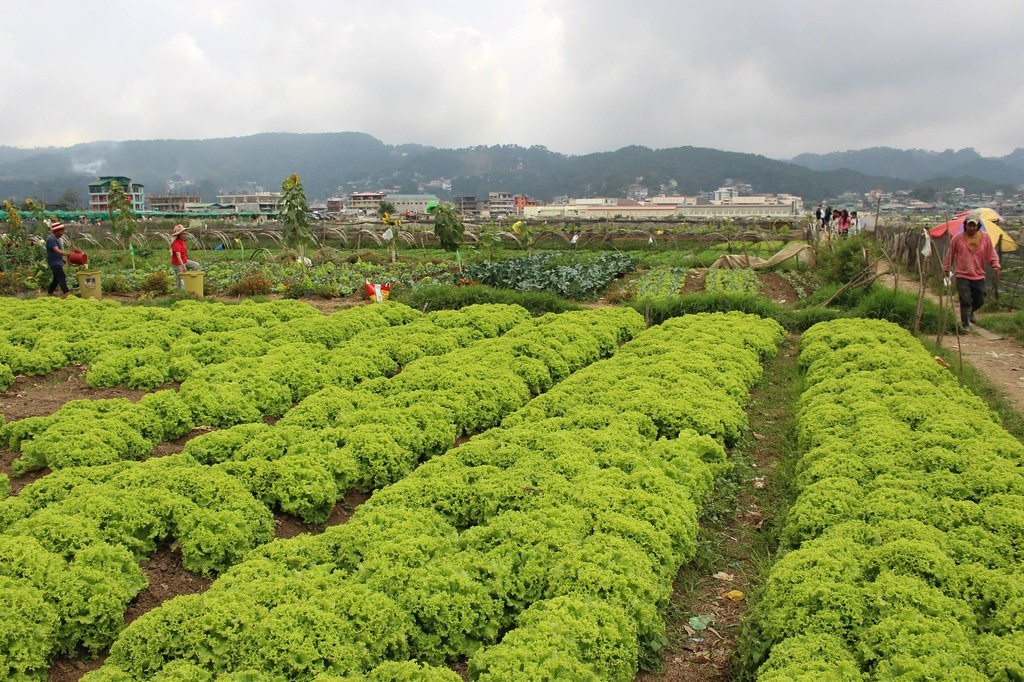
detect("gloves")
[943,271,949,278]
[993,268,1001,278]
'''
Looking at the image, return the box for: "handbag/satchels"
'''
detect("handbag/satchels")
[365,278,391,303]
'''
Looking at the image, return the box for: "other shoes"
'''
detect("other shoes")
[61,291,74,297]
[959,311,977,331]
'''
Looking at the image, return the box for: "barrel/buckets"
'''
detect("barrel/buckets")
[76,270,102,300]
[178,271,204,298]
[67,252,87,265]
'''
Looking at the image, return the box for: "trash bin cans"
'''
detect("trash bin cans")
[76,270,102,299]
[178,271,205,299]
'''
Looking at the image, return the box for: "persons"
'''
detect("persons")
[404,208,420,220]
[214,243,227,250]
[816,200,860,240]
[24,216,38,230]
[943,214,1001,334]
[131,215,152,223]
[220,213,237,222]
[46,222,73,298]
[171,224,199,289]
[93,217,101,226]
[44,216,51,231]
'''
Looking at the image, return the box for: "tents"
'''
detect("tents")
[0,209,288,221]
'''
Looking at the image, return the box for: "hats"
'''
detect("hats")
[172,224,188,236]
[51,222,65,232]
[965,214,980,225]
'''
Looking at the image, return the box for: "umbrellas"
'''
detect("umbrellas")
[927,208,1019,254]
